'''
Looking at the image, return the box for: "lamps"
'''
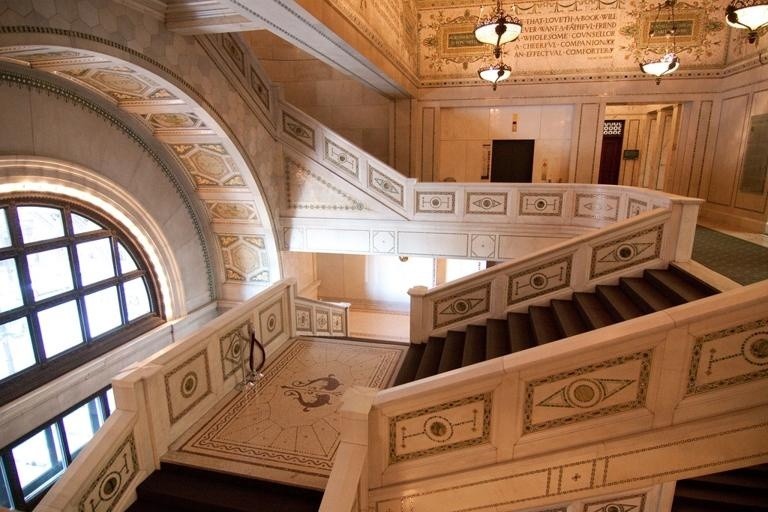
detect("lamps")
[725,0,768,44]
[639,0,680,85]
[473,0,522,90]
